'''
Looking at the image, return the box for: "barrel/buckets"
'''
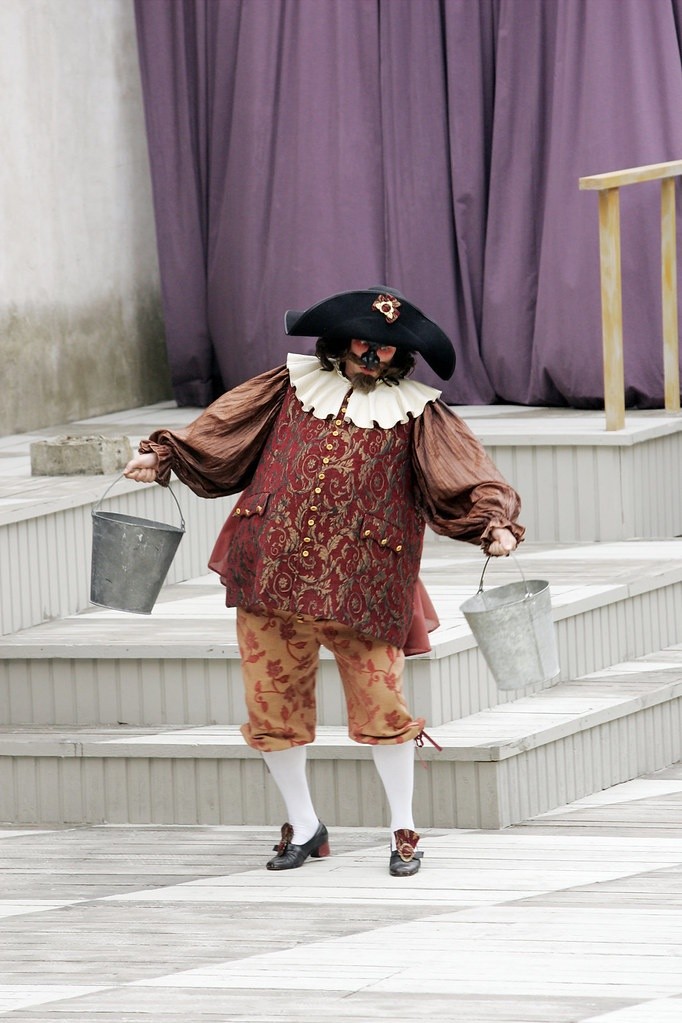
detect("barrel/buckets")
[89,473,186,615]
[458,549,560,691]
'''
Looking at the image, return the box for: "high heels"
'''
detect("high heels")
[389,828,423,877]
[266,818,331,871]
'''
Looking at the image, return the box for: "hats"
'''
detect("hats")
[283,284,457,381]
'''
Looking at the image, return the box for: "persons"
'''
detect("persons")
[127,282,529,878]
